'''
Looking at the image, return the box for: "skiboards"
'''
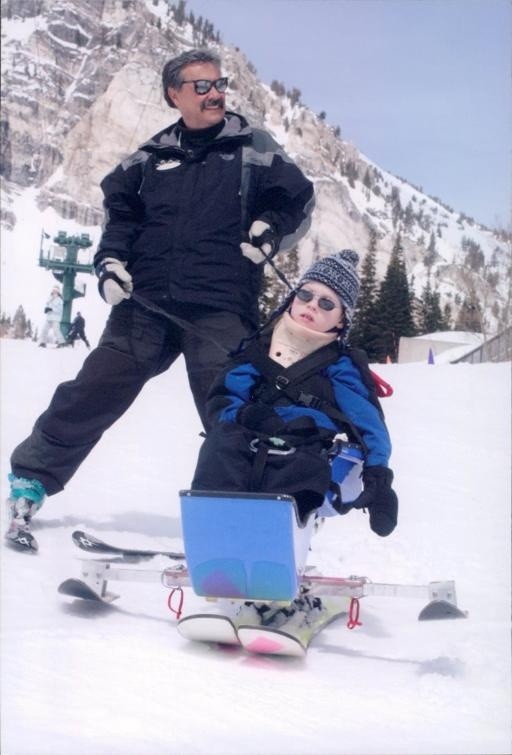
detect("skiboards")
[176,567,370,657]
[6,528,183,561]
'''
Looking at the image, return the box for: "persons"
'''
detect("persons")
[189,247,398,636]
[40,284,68,348]
[65,312,90,347]
[6,50,318,526]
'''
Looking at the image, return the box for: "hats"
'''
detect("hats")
[227,249,361,358]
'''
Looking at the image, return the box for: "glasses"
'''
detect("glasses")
[294,288,343,313]
[170,76,230,96]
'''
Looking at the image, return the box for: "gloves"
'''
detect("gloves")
[95,257,135,306]
[351,467,398,536]
[240,220,277,265]
[237,401,286,435]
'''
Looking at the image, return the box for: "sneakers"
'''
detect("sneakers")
[7,474,47,524]
